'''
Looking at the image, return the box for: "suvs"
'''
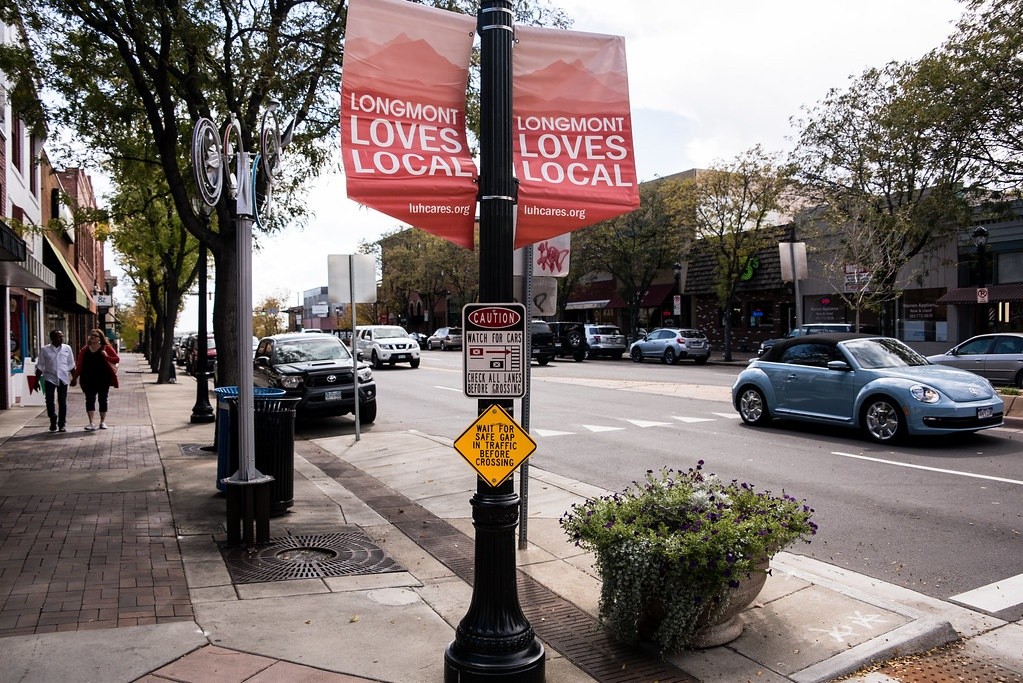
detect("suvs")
[546,322,587,362]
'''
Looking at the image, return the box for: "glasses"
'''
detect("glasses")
[91,334,99,338]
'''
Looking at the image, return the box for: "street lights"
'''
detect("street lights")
[671,262,683,326]
[972,226,989,335]
[189,193,216,422]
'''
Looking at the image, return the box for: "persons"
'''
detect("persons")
[70,329,119,430]
[33,330,76,432]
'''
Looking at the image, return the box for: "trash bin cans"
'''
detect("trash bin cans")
[212,387,303,519]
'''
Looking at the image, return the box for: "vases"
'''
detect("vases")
[693,562,769,648]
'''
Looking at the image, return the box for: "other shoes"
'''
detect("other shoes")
[100,422,107,429]
[85,423,95,430]
[50,420,56,430]
[59,427,66,432]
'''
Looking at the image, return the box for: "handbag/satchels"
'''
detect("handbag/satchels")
[103,345,118,374]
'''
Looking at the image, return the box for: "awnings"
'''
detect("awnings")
[44,235,97,314]
[937,285,1023,304]
[606,283,678,309]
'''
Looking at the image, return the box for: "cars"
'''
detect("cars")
[349,325,420,369]
[427,327,462,351]
[584,323,627,359]
[924,333,1023,390]
[629,327,712,365]
[408,334,428,350]
[731,332,1005,446]
[530,320,556,365]
[253,331,377,436]
[758,323,856,355]
[171,334,216,378]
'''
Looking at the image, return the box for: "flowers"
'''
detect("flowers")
[558,460,818,653]
